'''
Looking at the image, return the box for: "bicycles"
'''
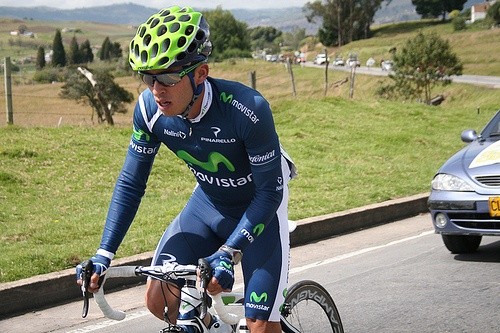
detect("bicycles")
[80,258,345,333]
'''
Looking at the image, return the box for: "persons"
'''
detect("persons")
[76,3,291,332]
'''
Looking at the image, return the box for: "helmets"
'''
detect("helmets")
[128,6,212,70]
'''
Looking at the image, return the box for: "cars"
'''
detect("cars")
[426,109,500,255]
[253,51,394,73]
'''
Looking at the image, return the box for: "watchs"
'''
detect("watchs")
[220,244,242,264]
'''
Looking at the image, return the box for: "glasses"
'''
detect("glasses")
[137,58,209,87]
